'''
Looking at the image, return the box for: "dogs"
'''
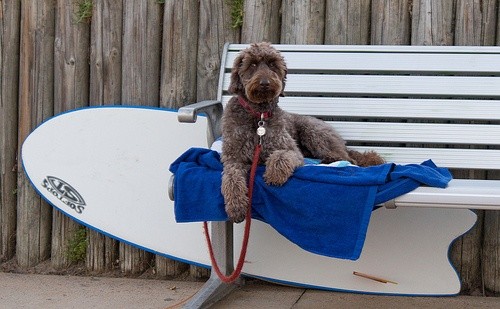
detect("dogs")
[222,41,386,224]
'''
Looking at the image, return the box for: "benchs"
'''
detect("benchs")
[168,41,500,309]
